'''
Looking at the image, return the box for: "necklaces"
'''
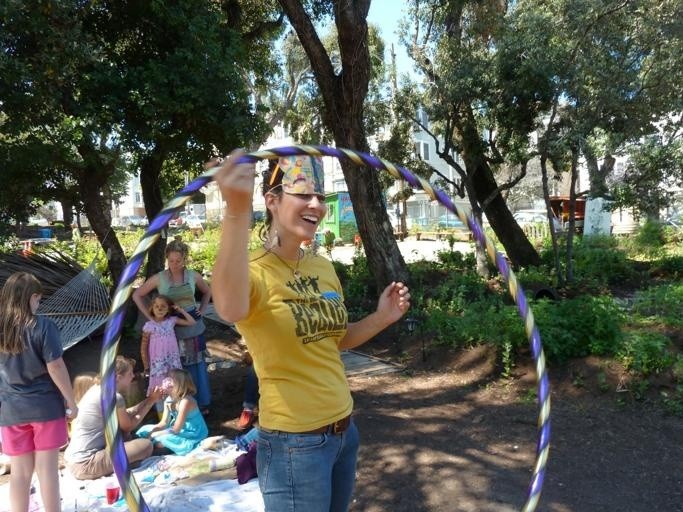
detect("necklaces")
[169,269,182,288]
[268,245,303,280]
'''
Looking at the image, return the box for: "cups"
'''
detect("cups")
[106,481,120,506]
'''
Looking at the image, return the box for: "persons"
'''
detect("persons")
[137,291,198,421]
[208,146,412,511]
[0,272,78,511]
[129,232,211,416]
[235,347,258,431]
[133,368,210,456]
[61,352,165,481]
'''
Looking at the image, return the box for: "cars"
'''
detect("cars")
[385,209,413,233]
[109,208,265,232]
[436,208,550,229]
[661,212,683,225]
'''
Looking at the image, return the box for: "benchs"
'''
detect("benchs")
[392,231,403,243]
[414,231,473,244]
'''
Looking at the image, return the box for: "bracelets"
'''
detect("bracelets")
[221,212,253,220]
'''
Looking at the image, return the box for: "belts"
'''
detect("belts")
[306,415,351,435]
